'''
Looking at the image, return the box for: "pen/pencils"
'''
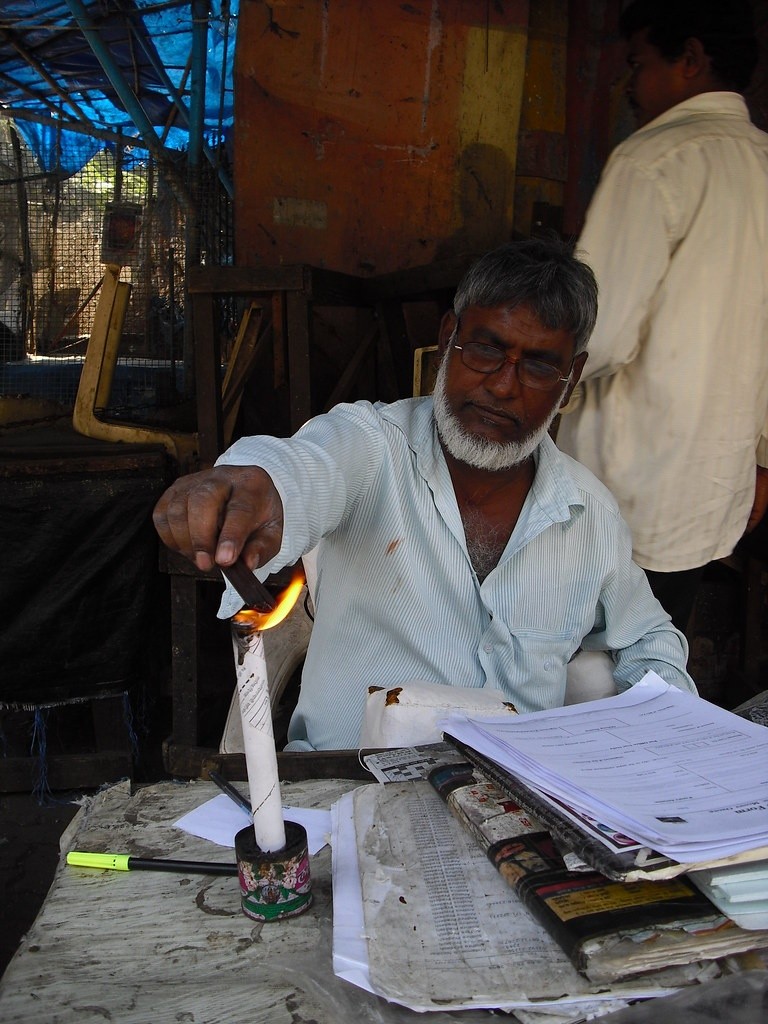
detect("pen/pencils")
[207,770,254,818]
[66,851,239,877]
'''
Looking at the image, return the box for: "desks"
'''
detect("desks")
[0,747,767,1024]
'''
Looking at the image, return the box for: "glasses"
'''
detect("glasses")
[457,314,576,385]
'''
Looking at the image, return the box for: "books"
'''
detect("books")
[426,669,768,990]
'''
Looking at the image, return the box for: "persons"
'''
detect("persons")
[151,239,701,759]
[547,0,768,713]
